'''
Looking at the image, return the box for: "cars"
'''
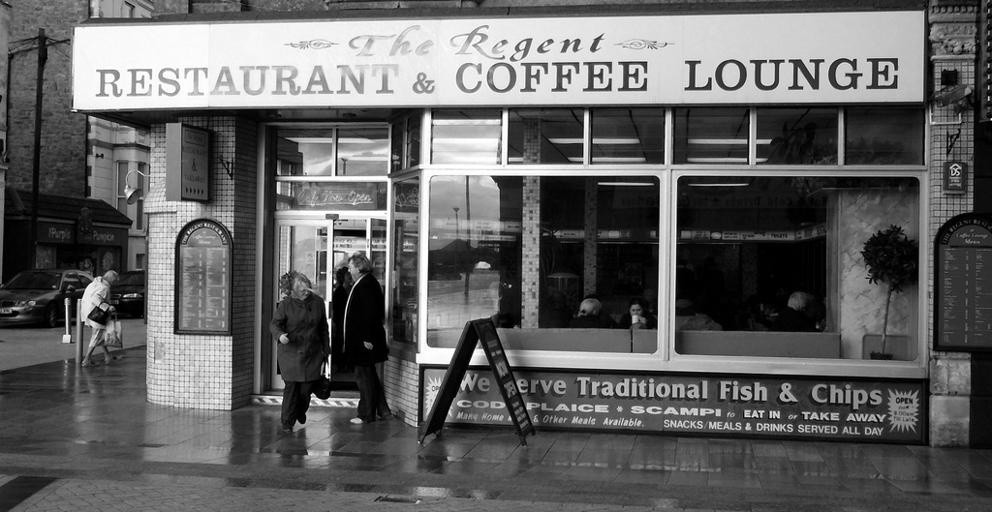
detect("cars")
[0,269,145,327]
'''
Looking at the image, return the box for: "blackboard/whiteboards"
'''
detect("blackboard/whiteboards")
[473,318,533,436]
[292,182,419,213]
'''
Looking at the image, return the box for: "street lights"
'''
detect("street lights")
[453,207,460,239]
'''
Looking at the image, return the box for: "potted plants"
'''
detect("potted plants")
[857,224,920,362]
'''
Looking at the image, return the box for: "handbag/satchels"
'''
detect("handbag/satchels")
[103,320,123,347]
[88,306,110,325]
[311,374,332,399]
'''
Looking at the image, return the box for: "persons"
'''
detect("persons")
[80,268,127,368]
[333,267,353,374]
[773,291,819,330]
[567,288,657,328]
[267,275,331,433]
[341,256,394,428]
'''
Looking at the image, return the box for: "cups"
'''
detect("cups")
[632,314,639,323]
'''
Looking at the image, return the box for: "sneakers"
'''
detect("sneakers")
[349,410,392,425]
[81,356,116,369]
[297,414,306,425]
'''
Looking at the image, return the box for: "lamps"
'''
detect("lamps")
[124,168,164,206]
